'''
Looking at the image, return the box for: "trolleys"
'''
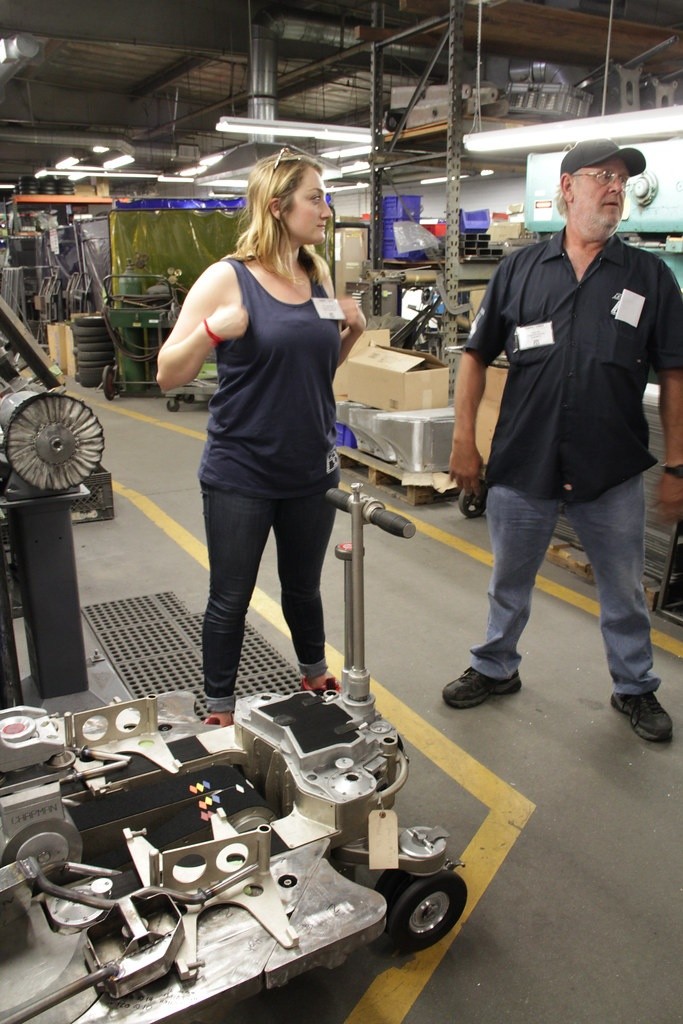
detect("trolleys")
[0,482,471,1023]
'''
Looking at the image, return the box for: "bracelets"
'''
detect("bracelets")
[664,463,683,478]
[204,319,224,344]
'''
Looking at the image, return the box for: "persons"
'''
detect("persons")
[442,132,683,744]
[157,147,367,727]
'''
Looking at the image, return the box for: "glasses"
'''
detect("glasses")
[273,144,323,171]
[570,169,631,192]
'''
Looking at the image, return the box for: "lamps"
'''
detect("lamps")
[33,0,394,192]
[463,0,683,152]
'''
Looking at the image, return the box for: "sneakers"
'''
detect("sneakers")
[442,667,521,710]
[300,676,342,697]
[611,689,673,741]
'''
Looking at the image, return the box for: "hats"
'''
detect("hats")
[561,139,647,177]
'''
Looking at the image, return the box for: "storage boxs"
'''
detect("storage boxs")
[70,462,115,523]
[384,196,490,259]
[332,329,389,401]
[347,345,449,412]
[475,365,509,464]
